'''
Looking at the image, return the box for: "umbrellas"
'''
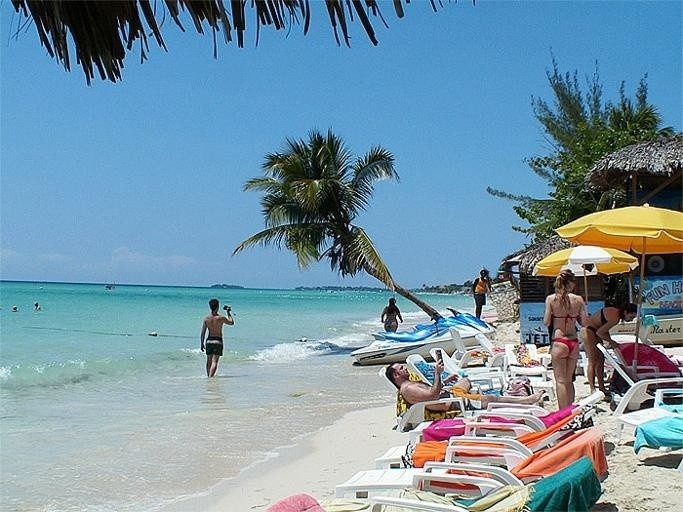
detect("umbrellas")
[531,244,639,313]
[553,203,683,375]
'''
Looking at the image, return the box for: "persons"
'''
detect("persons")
[201,299,234,378]
[386,358,544,411]
[580,303,637,395]
[473,269,488,320]
[381,298,403,332]
[34,301,39,311]
[543,269,589,410]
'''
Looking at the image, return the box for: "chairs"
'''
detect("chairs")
[379,328,550,405]
[267,391,616,512]
[592,314,683,469]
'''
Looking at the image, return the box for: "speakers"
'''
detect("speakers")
[645,255,669,276]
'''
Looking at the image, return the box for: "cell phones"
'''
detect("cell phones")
[436,350,443,365]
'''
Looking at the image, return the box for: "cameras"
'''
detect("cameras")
[223,305,228,310]
